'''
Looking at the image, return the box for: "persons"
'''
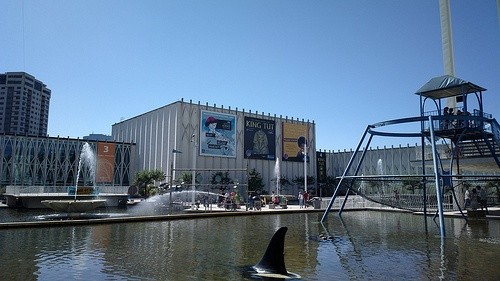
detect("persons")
[217,189,278,211]
[296,136,308,159]
[253,122,269,154]
[298,191,309,209]
[203,116,234,154]
[442,107,472,129]
[464,185,488,211]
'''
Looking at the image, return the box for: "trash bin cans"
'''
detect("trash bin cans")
[254,201,261,211]
[269,202,275,208]
[314,197,320,209]
